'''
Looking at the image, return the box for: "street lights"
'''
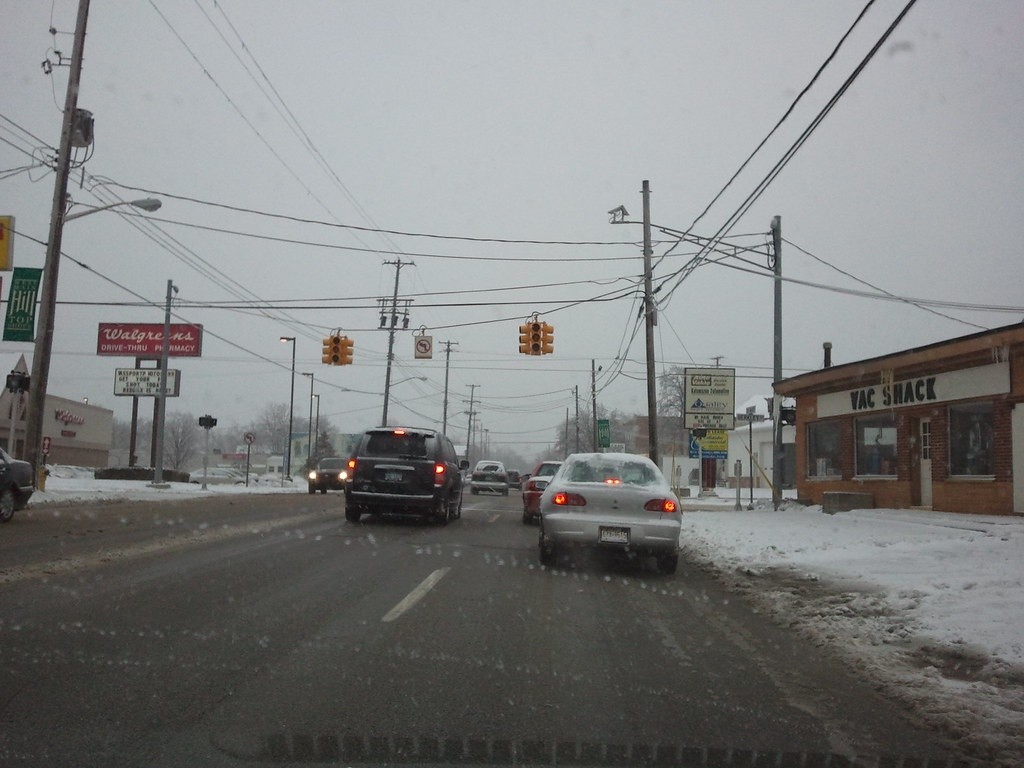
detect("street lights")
[381,375,430,426]
[302,372,313,459]
[21,197,165,468]
[313,395,320,455]
[279,336,296,481]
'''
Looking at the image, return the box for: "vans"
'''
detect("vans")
[343,425,470,523]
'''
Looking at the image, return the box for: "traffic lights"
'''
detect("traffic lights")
[541,323,554,354]
[519,324,532,355]
[5,372,23,393]
[329,335,342,365]
[529,321,542,355]
[342,338,355,365]
[322,336,334,364]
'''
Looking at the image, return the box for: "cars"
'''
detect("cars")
[0,449,35,522]
[465,474,473,486]
[535,449,681,573]
[188,467,283,485]
[520,458,566,523]
[307,456,351,494]
[470,460,507,495]
[508,469,522,491]
